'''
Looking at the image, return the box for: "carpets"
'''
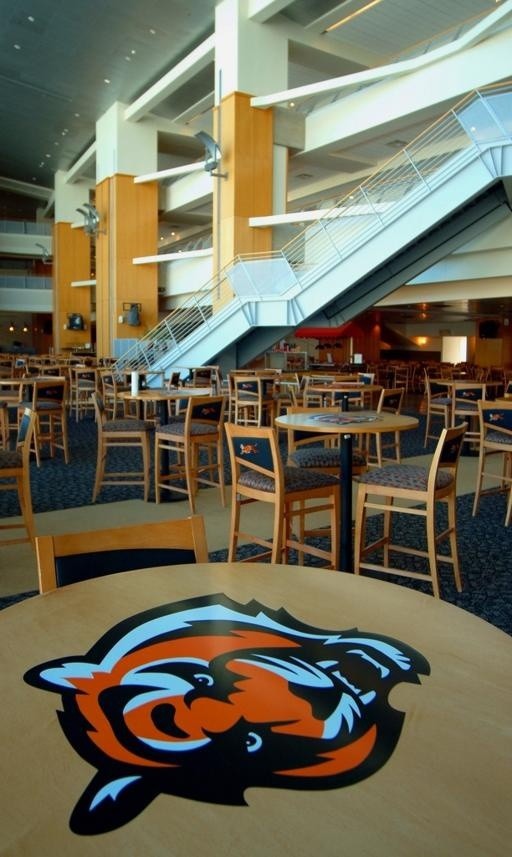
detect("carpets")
[0,485,512,634]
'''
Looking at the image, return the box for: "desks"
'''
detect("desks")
[0,562,512,857]
